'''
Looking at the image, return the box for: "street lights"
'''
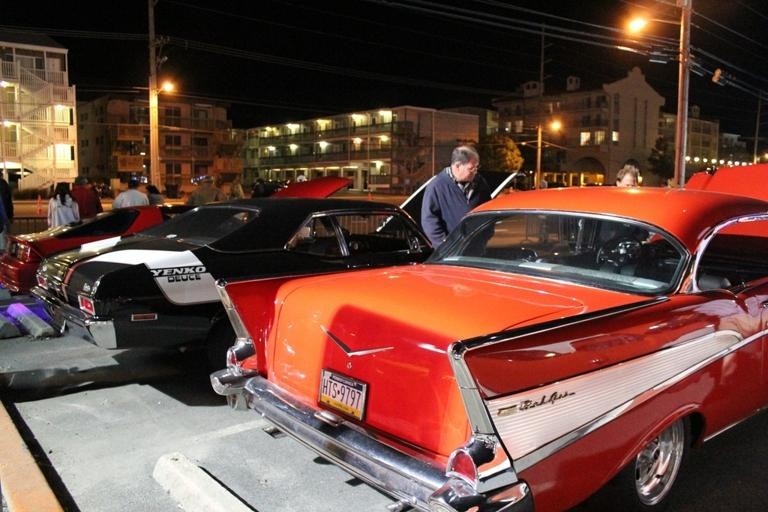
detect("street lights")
[622,0,695,184]
[145,75,180,189]
[532,115,566,187]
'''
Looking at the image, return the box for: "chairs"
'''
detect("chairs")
[342,227,409,254]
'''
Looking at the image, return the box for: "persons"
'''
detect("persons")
[420,143,494,258]
[507,174,566,192]
[0,171,15,233]
[0,197,11,251]
[47,172,307,231]
[594,164,653,255]
